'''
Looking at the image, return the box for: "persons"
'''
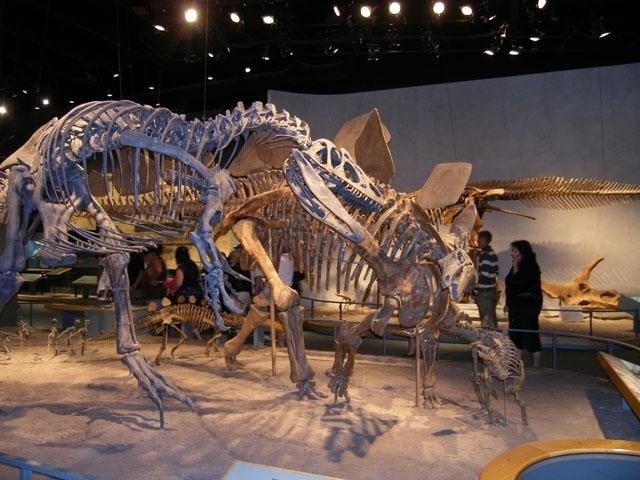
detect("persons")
[98,237,307,346]
[473,231,500,330]
[504,240,543,369]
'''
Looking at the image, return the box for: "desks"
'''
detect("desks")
[16,267,99,298]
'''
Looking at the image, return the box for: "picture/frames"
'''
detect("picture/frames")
[219,459,340,480]
